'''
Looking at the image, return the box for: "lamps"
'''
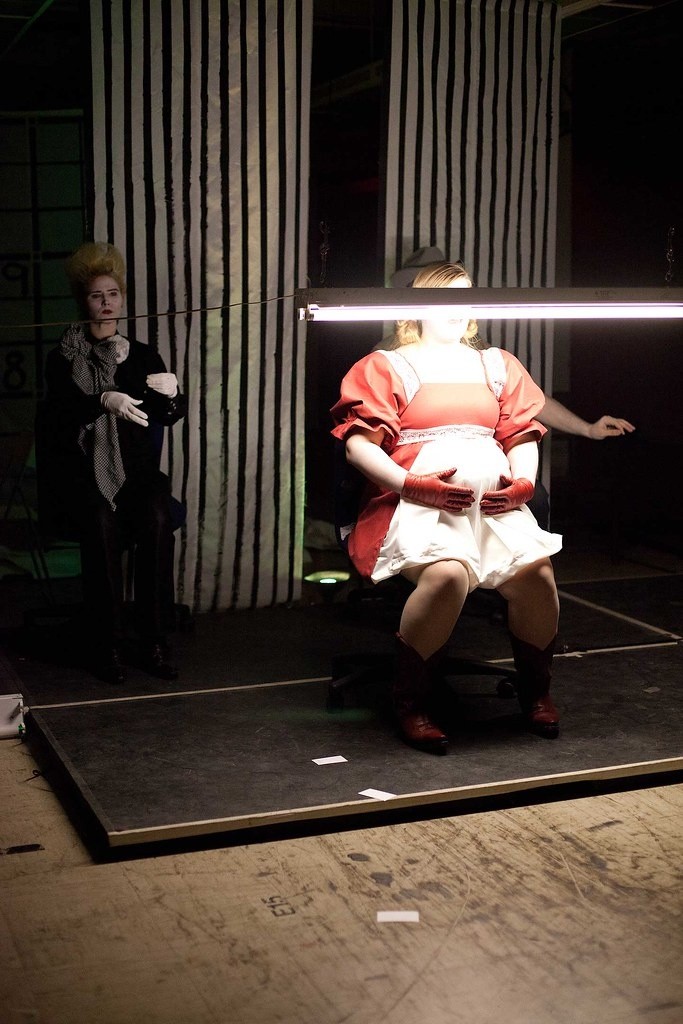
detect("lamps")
[295,288,683,319]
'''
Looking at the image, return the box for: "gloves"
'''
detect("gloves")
[402,470,475,513]
[101,390,150,427]
[480,476,535,515]
[146,373,180,399]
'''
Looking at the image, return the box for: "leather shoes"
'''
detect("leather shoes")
[90,641,126,683]
[144,636,171,674]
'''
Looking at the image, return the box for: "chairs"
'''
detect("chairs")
[34,403,185,640]
[327,443,523,707]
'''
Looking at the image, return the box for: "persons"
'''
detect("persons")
[33,242,189,685]
[329,262,638,752]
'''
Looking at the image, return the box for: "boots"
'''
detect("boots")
[511,624,561,731]
[394,631,451,748]
